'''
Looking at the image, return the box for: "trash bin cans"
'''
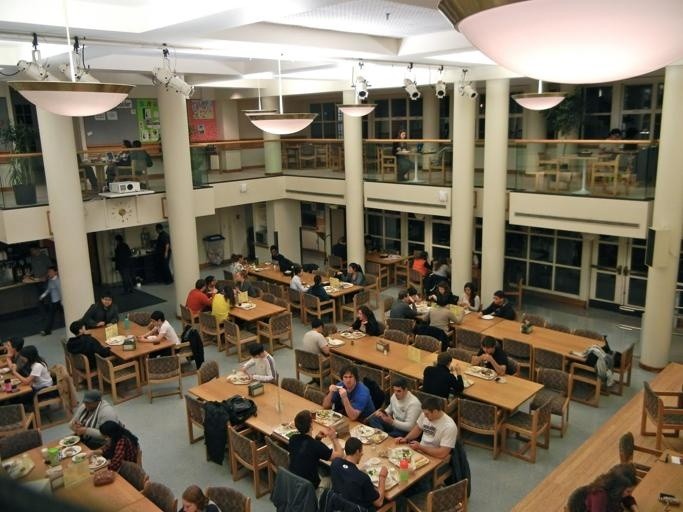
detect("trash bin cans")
[203,234,225,266]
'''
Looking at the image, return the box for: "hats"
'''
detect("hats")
[83,389,101,403]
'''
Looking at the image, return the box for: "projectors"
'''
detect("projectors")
[109,181,140,193]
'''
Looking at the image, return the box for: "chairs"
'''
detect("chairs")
[327,251,527,310]
[612,377,682,512]
[175,251,379,364]
[363,144,452,183]
[290,320,575,467]
[381,289,640,409]
[282,138,345,172]
[527,141,649,198]
[2,427,252,512]
[181,359,471,512]
[79,149,149,201]
[1,311,192,427]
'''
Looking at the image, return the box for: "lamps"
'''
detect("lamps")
[1,33,65,81]
[513,79,565,110]
[6,4,136,117]
[460,66,478,99]
[403,62,420,100]
[439,0,683,83]
[436,65,448,99]
[334,57,378,117]
[243,52,318,135]
[152,44,195,99]
[55,34,102,83]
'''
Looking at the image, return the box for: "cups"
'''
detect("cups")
[350,340,353,345]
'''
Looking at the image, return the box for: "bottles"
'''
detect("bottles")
[39,444,57,467]
[4,379,11,392]
[123,315,128,330]
[0,375,4,388]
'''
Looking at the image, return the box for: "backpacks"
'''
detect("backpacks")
[225,394,257,422]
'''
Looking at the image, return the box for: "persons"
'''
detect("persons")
[21,244,62,336]
[303,306,381,386]
[121,140,131,160]
[581,463,638,511]
[184,246,370,328]
[106,140,152,184]
[599,129,621,157]
[332,236,347,261]
[2,335,222,511]
[68,225,182,371]
[422,352,464,401]
[389,251,517,375]
[392,129,412,181]
[77,154,97,192]
[242,344,276,382]
[288,410,344,489]
[323,364,458,510]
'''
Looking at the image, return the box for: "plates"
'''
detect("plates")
[105,335,125,345]
[89,456,106,468]
[0,367,9,374]
[226,374,252,385]
[404,300,495,321]
[61,446,81,457]
[254,268,264,272]
[234,302,256,310]
[71,453,86,461]
[328,339,343,346]
[447,365,497,388]
[59,434,80,447]
[321,281,353,294]
[283,408,429,483]
[352,331,362,336]
[341,332,350,337]
[9,378,20,386]
[2,459,21,475]
[22,277,38,283]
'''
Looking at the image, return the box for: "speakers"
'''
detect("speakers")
[644,227,670,268]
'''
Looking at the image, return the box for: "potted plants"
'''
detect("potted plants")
[0,121,37,204]
